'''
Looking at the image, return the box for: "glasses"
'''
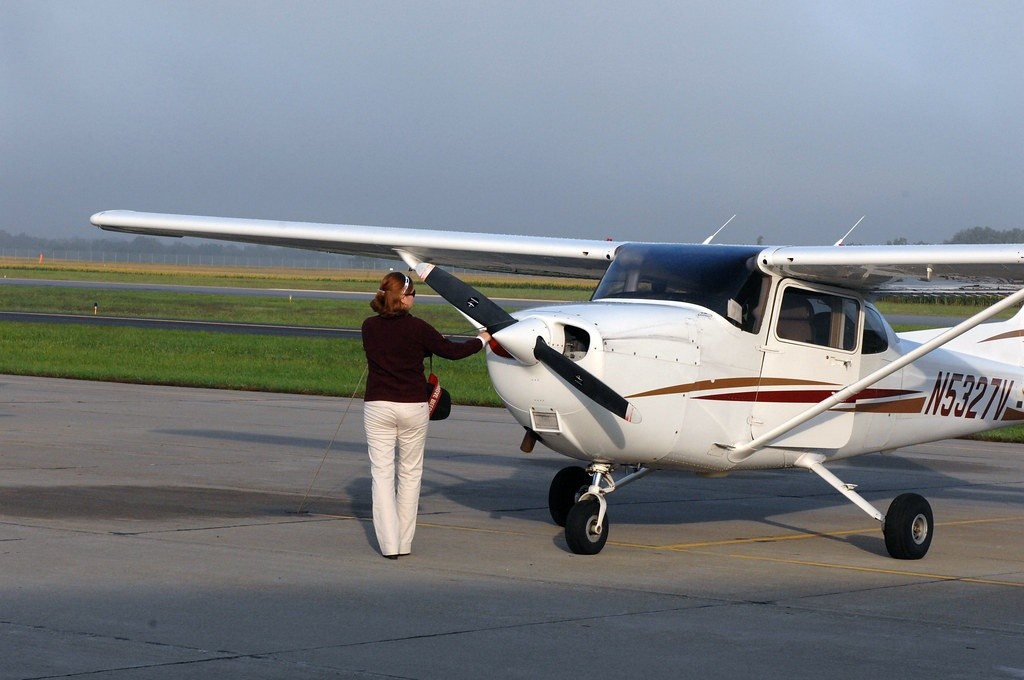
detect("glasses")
[403,289,415,297]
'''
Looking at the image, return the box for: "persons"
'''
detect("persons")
[361,272,492,560]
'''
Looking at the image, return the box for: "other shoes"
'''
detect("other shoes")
[383,553,399,560]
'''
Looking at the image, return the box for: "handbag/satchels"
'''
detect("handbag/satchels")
[426,374,451,420]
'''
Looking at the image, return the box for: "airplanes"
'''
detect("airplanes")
[88,208,1024,564]
